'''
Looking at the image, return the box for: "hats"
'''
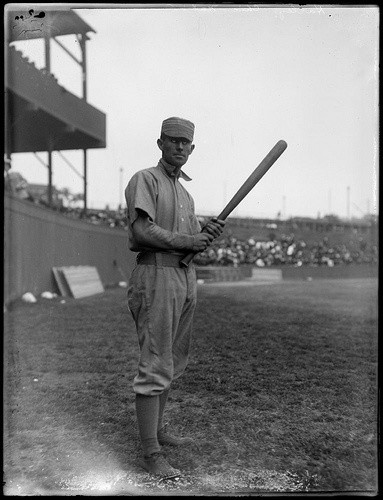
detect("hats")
[161,117,195,142]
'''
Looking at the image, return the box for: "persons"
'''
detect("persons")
[124,117,227,480]
[197,233,378,268]
[57,203,131,233]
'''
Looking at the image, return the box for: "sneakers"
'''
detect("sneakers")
[158,426,193,448]
[138,453,180,481]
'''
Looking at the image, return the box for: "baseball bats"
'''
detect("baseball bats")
[180,140,287,268]
